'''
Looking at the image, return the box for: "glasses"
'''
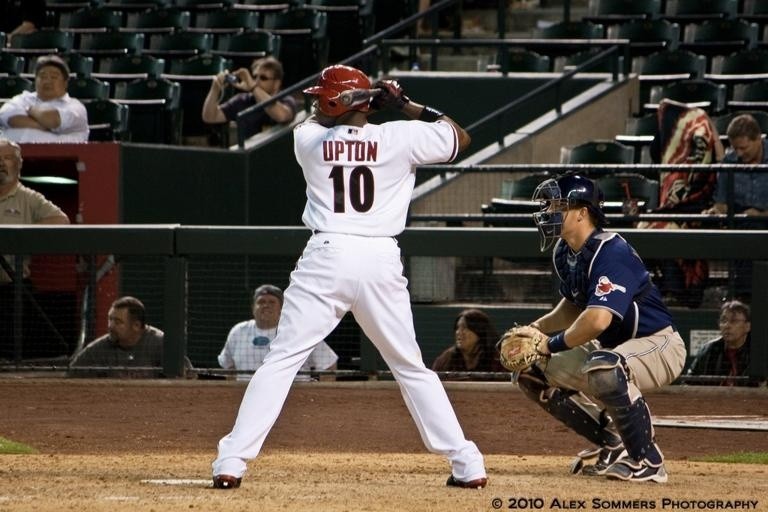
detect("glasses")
[252,74,276,81]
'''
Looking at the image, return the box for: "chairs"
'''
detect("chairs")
[0,0,766,306]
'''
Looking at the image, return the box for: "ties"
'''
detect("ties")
[721,346,741,387]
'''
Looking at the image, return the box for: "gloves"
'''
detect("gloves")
[372,79,412,117]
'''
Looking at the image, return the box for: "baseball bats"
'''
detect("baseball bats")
[342,87,386,107]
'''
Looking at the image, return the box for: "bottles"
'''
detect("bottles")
[622,198,639,217]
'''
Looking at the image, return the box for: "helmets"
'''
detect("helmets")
[302,64,375,118]
[530,173,609,253]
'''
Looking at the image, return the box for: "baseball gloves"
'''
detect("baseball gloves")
[497,325,551,371]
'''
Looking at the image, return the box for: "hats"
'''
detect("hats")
[252,283,284,303]
[35,53,69,81]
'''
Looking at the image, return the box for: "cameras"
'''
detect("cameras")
[224,73,237,84]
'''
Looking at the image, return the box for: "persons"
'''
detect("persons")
[432,308,512,372]
[496,173,689,484]
[201,56,296,151]
[700,114,768,303]
[0,53,91,144]
[67,295,198,381]
[212,65,488,489]
[679,297,754,387]
[0,0,46,48]
[632,98,726,308]
[218,284,341,382]
[0,138,73,361]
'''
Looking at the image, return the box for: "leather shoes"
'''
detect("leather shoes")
[210,473,244,491]
[444,475,488,490]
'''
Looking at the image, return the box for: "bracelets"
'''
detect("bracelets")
[250,85,258,92]
[547,331,573,352]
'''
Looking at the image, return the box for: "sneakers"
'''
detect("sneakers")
[605,438,669,485]
[581,437,629,476]
